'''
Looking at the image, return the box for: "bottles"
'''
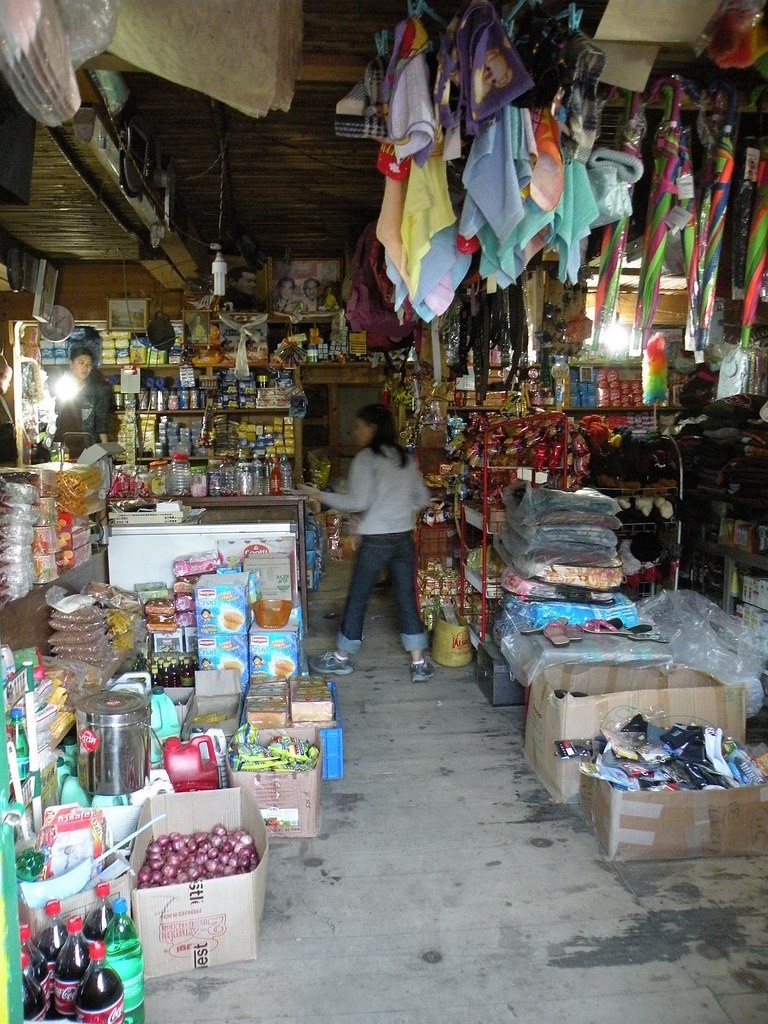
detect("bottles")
[74,941,126,1024]
[108,449,293,499]
[147,686,181,770]
[21,949,46,1022]
[20,924,52,1020]
[54,915,93,1022]
[112,382,207,410]
[0,643,44,884]
[132,653,200,689]
[83,882,113,947]
[23,438,69,465]
[102,898,146,1023]
[35,899,67,1021]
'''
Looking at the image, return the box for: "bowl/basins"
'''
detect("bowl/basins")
[252,599,293,629]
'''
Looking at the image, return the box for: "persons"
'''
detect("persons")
[297,404,435,682]
[49,347,110,546]
[184,315,207,343]
[218,265,323,313]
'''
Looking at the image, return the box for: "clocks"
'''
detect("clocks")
[38,304,74,343]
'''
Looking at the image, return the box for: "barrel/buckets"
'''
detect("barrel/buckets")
[181,727,228,788]
[163,736,218,793]
[106,672,151,697]
[430,616,473,668]
[74,688,153,796]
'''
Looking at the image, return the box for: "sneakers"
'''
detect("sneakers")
[411,659,434,682]
[309,652,354,675]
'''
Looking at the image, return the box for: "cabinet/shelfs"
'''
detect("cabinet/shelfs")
[445,363,686,410]
[593,434,683,598]
[0,660,42,1024]
[415,412,569,650]
[42,363,302,488]
[679,534,768,725]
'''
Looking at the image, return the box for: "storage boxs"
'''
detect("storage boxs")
[736,575,768,633]
[455,369,507,406]
[17,552,345,979]
[520,663,747,805]
[580,752,767,862]
[477,643,524,706]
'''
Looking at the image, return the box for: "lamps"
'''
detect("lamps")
[60,432,126,471]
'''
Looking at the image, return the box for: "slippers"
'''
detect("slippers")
[628,633,670,644]
[520,617,568,635]
[583,619,633,635]
[542,622,569,647]
[567,623,582,641]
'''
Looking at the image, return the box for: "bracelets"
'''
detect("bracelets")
[309,489,321,499]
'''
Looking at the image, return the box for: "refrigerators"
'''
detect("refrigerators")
[105,514,302,658]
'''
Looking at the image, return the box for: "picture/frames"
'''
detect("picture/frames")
[32,258,59,323]
[106,298,150,332]
[210,254,344,314]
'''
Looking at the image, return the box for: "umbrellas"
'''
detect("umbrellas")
[592,76,768,353]
